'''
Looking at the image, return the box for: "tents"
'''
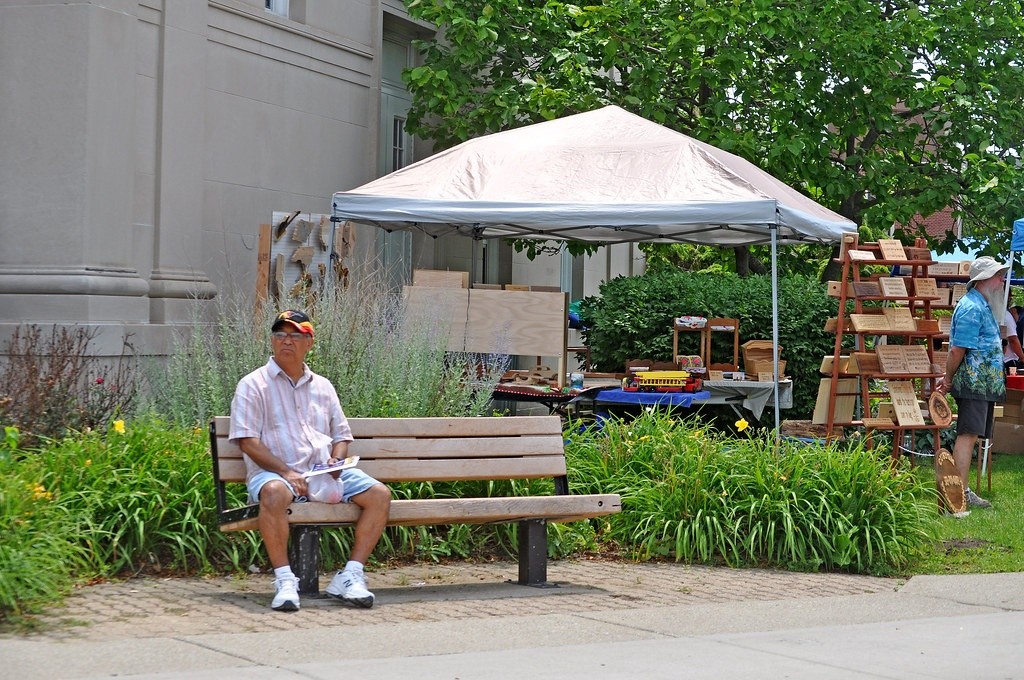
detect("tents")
[321,104,858,456]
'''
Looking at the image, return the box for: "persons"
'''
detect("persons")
[1000,285,1024,375]
[227,309,391,614]
[935,255,1011,508]
[1008,306,1024,352]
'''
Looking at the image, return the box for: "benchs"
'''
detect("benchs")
[210,411,623,594]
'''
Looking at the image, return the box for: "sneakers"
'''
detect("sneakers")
[326,569,374,609]
[270,573,300,611]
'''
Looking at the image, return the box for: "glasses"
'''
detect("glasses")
[995,272,1006,278]
[274,332,307,342]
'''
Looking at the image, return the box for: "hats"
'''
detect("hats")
[966,256,1010,292]
[928,391,952,426]
[270,310,314,337]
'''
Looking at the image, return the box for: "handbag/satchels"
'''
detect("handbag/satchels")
[308,473,344,504]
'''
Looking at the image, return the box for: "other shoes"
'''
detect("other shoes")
[965,487,990,507]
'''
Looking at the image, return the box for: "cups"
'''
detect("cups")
[1009,367,1017,376]
[571,374,584,389]
